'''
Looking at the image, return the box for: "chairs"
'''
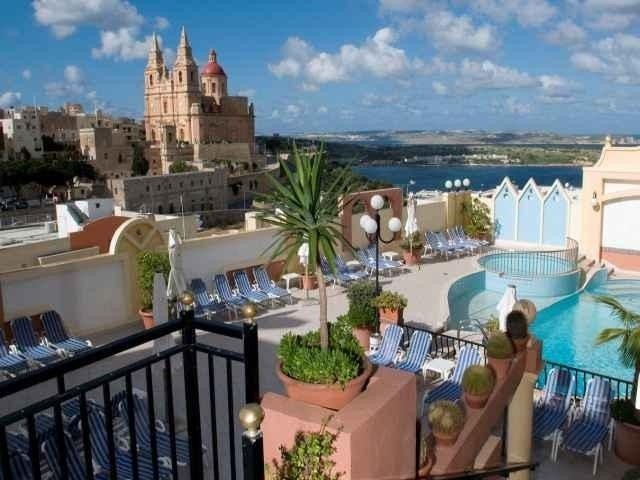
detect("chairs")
[190,223,490,322]
[393,331,432,375]
[555,378,619,474]
[366,325,404,368]
[531,368,577,462]
[425,346,485,408]
[0,311,208,480]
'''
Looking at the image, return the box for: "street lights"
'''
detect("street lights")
[360,195,401,294]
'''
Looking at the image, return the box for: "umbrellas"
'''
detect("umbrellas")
[167,229,188,319]
[404,190,418,264]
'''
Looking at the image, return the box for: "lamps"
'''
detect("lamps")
[445,177,471,193]
[358,194,402,298]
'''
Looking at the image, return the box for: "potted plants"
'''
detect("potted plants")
[428,399,464,447]
[461,197,494,241]
[462,365,497,409]
[508,310,533,352]
[482,328,515,376]
[244,136,374,412]
[348,295,375,350]
[134,250,170,329]
[588,291,640,467]
[414,417,436,478]
[372,290,405,333]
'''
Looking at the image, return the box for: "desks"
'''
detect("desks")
[423,358,454,386]
